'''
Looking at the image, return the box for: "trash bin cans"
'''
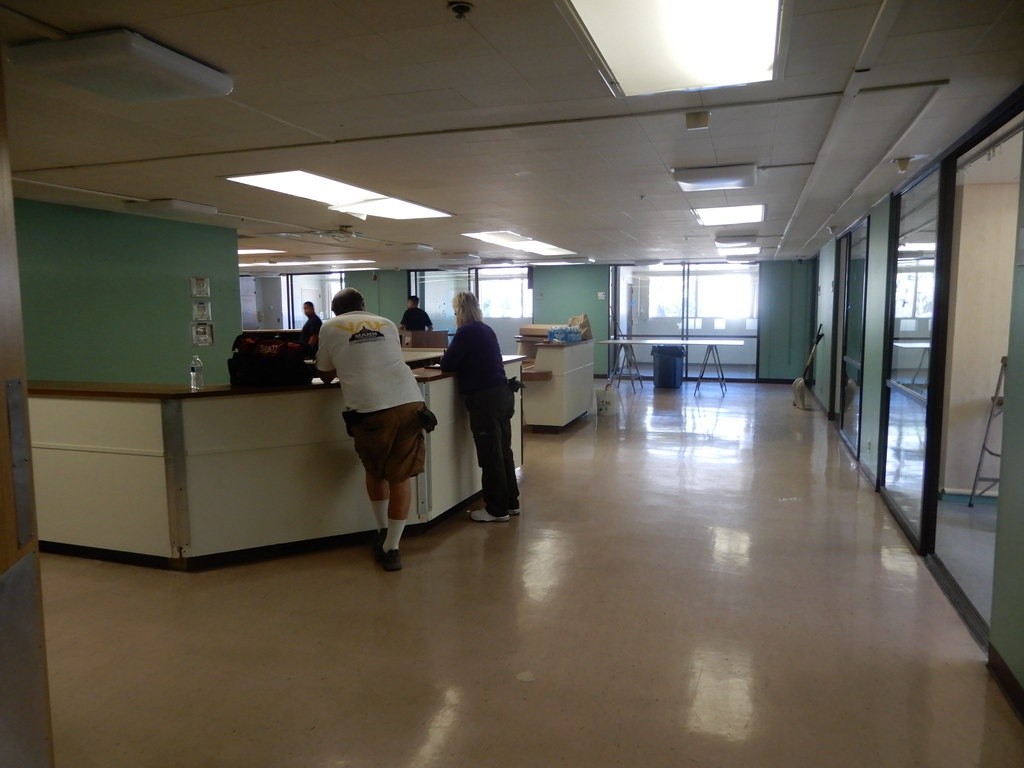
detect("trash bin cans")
[650,345,687,389]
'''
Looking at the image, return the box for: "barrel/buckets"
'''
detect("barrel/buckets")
[595,387,617,416]
[652,346,685,388]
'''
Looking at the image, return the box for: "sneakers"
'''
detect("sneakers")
[377,527,388,545]
[374,548,401,571]
[470,509,510,522]
[507,508,521,515]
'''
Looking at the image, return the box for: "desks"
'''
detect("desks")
[596,340,745,398]
[893,342,930,386]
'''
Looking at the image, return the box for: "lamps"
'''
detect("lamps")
[385,243,433,253]
[671,162,759,192]
[3,28,234,103]
[553,0,796,99]
[123,197,218,219]
[431,252,481,266]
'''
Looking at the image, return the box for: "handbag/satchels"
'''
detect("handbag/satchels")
[569,314,593,341]
[596,384,619,417]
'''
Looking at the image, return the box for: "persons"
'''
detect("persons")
[440,292,520,522]
[302,302,322,331]
[400,296,433,332]
[314,288,426,572]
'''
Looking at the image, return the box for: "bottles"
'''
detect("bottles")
[191,355,203,389]
[548,326,582,342]
